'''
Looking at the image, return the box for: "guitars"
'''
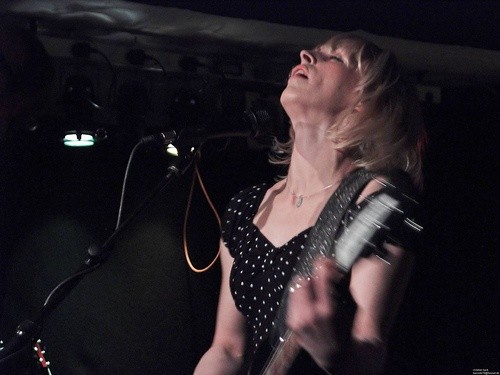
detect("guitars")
[264,184,421,374]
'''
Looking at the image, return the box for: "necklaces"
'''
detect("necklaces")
[284,175,342,207]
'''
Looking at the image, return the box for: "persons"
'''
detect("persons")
[190,31,441,375]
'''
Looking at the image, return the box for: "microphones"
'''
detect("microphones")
[165,108,272,146]
